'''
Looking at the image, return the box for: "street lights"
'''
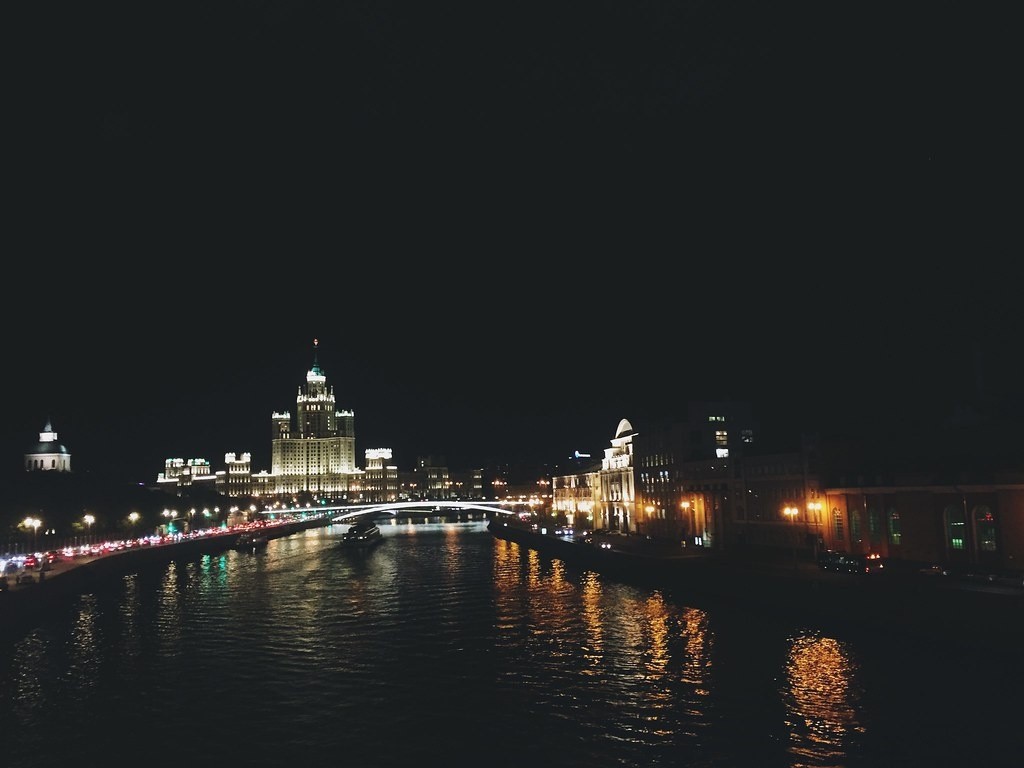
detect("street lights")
[25,514,42,552]
[783,504,801,570]
[806,501,824,562]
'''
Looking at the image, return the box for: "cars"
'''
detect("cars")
[919,564,951,576]
[817,550,886,575]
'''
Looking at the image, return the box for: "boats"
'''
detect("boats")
[233,530,270,547]
[338,520,384,547]
